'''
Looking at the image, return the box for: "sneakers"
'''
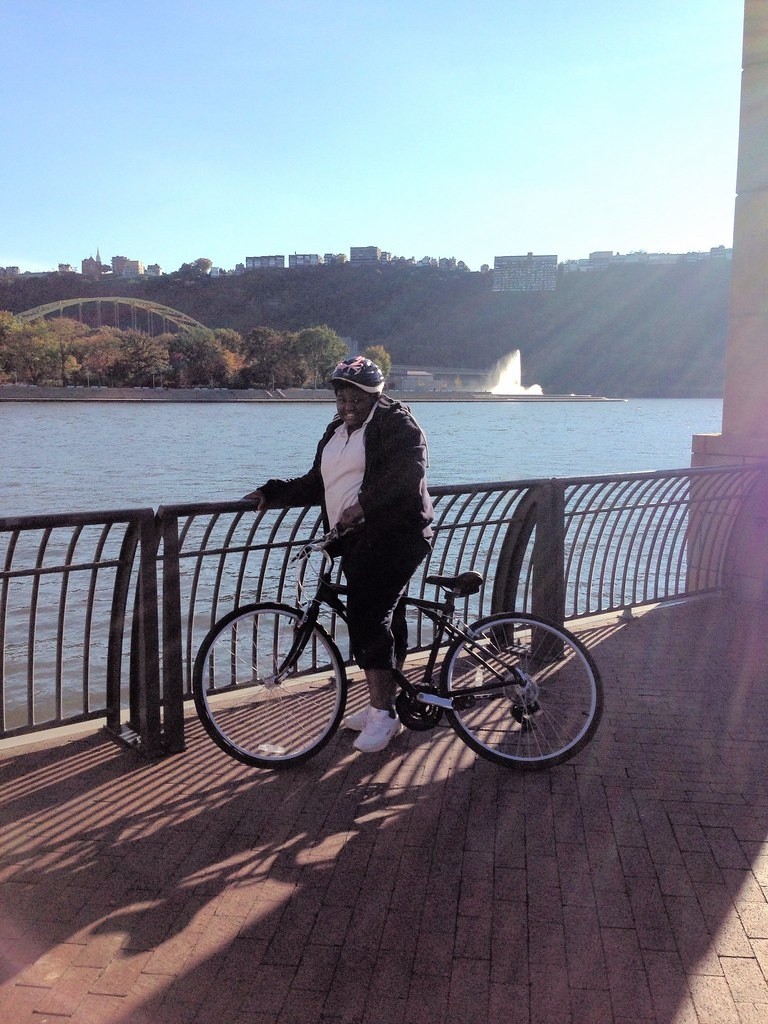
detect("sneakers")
[344,704,399,730]
[354,705,402,754]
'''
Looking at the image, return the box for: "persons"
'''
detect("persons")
[242,355,434,755]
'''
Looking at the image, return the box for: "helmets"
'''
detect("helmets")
[327,355,385,403]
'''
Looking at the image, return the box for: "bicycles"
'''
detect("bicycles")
[191,516,605,772]
